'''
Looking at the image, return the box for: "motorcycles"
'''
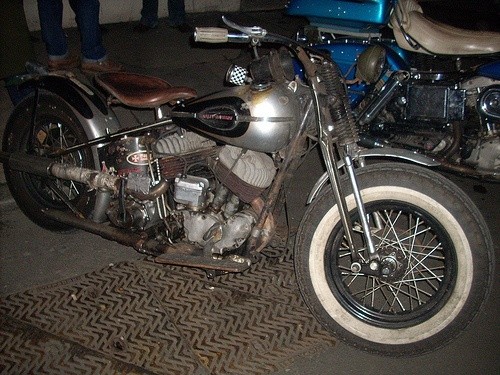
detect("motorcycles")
[283,0,500,182]
[0,14,497,359]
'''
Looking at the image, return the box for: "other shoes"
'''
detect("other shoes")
[169,22,192,33]
[44,57,78,71]
[80,59,125,74]
[133,24,160,33]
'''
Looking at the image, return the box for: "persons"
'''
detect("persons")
[37,0,126,74]
[133,0,194,34]
